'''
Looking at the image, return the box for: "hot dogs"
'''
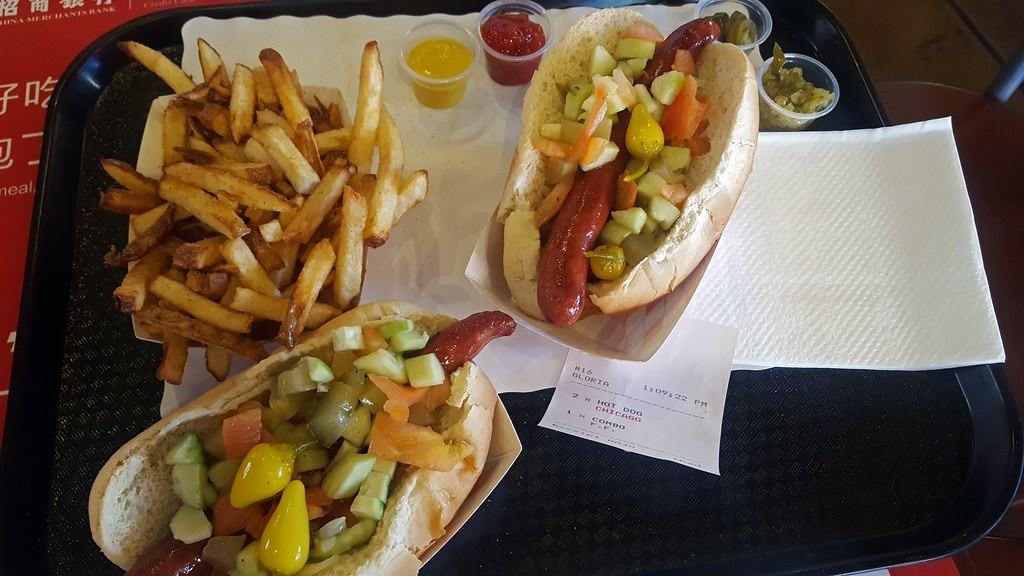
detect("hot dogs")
[89,301,516,576]
[496,8,760,326]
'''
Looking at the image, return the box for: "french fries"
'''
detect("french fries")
[101,38,428,386]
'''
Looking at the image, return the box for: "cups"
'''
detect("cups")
[760,55,840,133]
[695,0,773,53]
[399,18,480,107]
[477,0,555,84]
